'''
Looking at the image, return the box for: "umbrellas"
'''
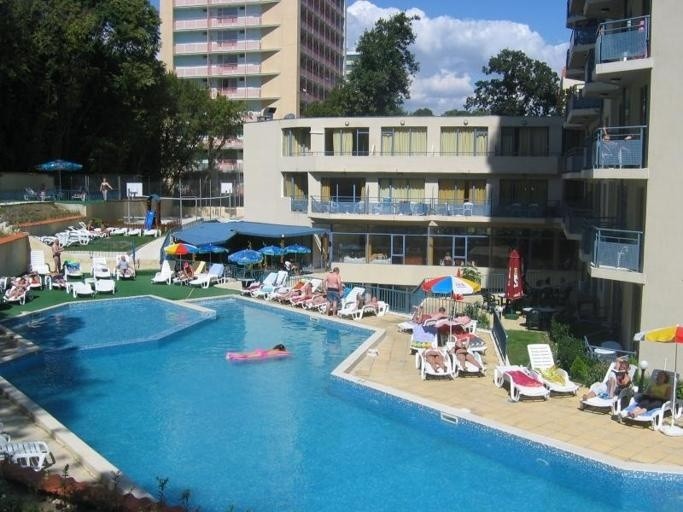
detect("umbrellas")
[505,250,525,301]
[35,159,83,192]
[228,249,263,279]
[197,243,230,264]
[163,243,200,271]
[284,243,312,262]
[258,244,288,256]
[421,275,481,340]
[634,325,683,437]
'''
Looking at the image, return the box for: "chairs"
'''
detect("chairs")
[150,260,226,289]
[599,238,634,270]
[24,188,87,203]
[36,221,163,249]
[293,196,474,215]
[598,132,632,169]
[227,255,389,321]
[493,343,582,403]
[0,422,11,443]
[0,441,51,472]
[582,360,681,430]
[1,251,134,306]
[398,307,487,382]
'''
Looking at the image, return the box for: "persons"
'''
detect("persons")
[180,263,198,280]
[86,220,95,231]
[116,256,133,276]
[101,221,111,234]
[227,344,289,360]
[37,184,48,202]
[99,178,114,202]
[583,358,630,401]
[413,304,453,323]
[422,345,447,372]
[443,252,452,265]
[279,259,379,318]
[52,239,64,273]
[7,270,68,299]
[448,339,483,372]
[628,371,672,419]
